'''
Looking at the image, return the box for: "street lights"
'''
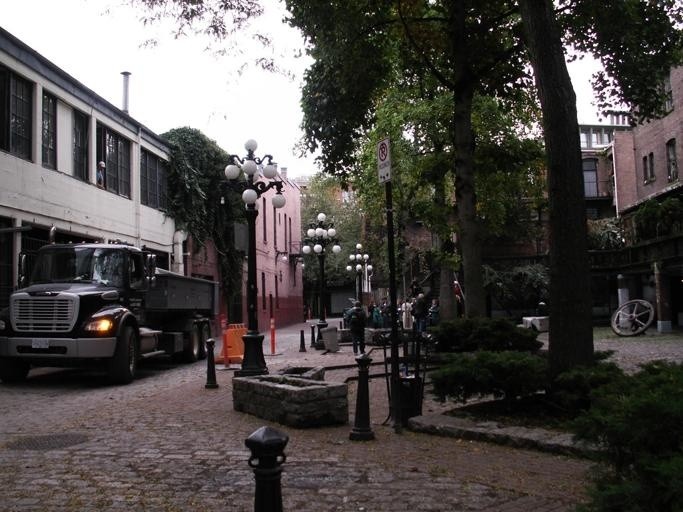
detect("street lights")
[344,242,373,305]
[222,138,286,376]
[301,209,342,353]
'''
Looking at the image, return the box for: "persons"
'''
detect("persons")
[344,281,461,355]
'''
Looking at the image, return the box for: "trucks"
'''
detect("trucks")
[0,223,216,383]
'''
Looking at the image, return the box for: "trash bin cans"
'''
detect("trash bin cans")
[379,330,438,426]
[320,326,339,353]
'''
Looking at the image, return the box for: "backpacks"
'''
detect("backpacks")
[348,309,364,333]
